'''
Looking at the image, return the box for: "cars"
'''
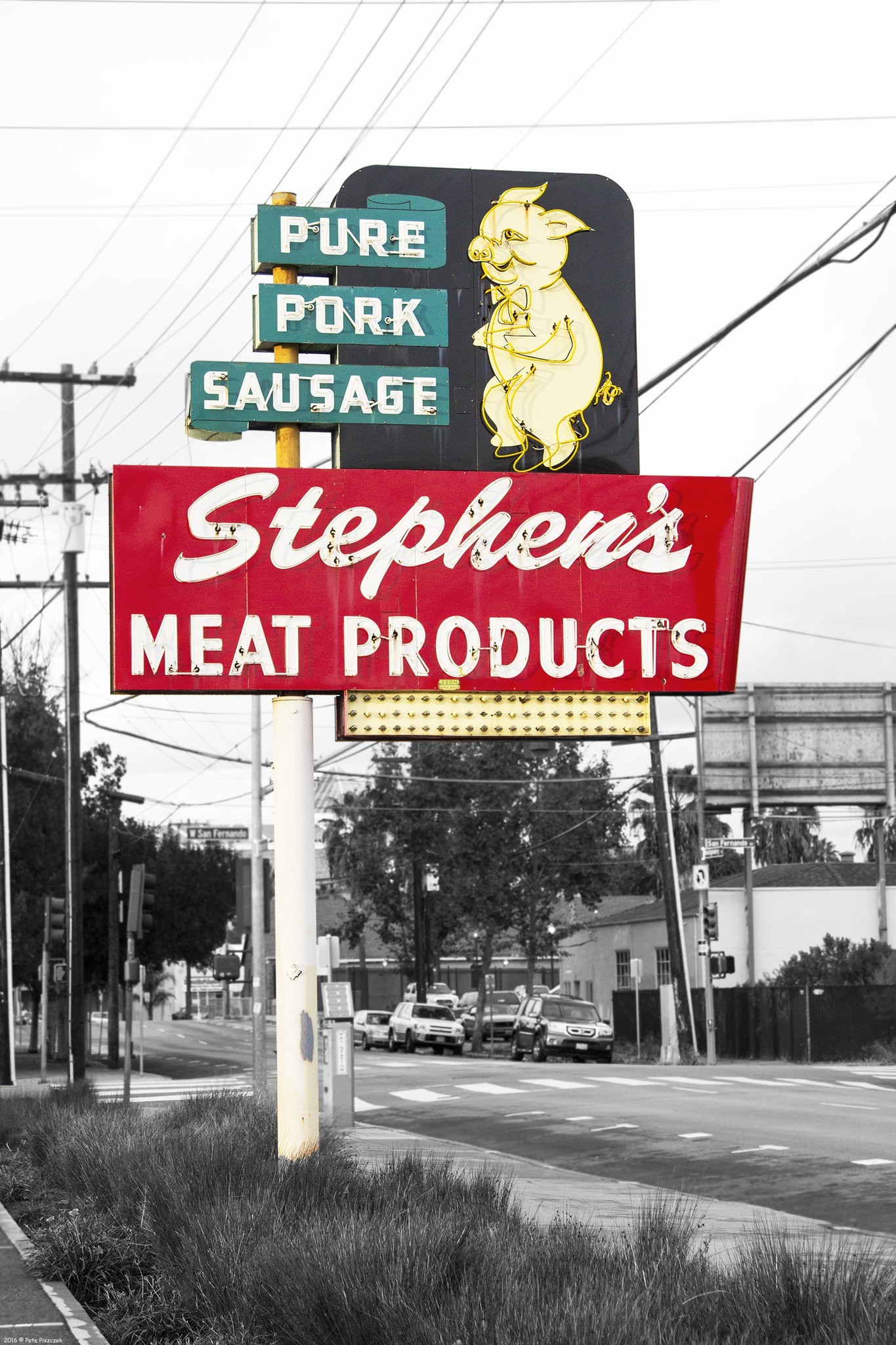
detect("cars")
[515,985,556,1004]
[352,1010,398,1051]
[463,1005,517,1042]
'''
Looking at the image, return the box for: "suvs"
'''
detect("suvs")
[404,982,460,1010]
[511,994,615,1064]
[458,990,522,1013]
[390,1002,466,1056]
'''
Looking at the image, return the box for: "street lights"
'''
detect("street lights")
[548,923,556,990]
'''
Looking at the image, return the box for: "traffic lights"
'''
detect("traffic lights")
[702,902,720,940]
[712,956,735,974]
[54,964,67,983]
[43,897,68,944]
[128,863,158,940]
